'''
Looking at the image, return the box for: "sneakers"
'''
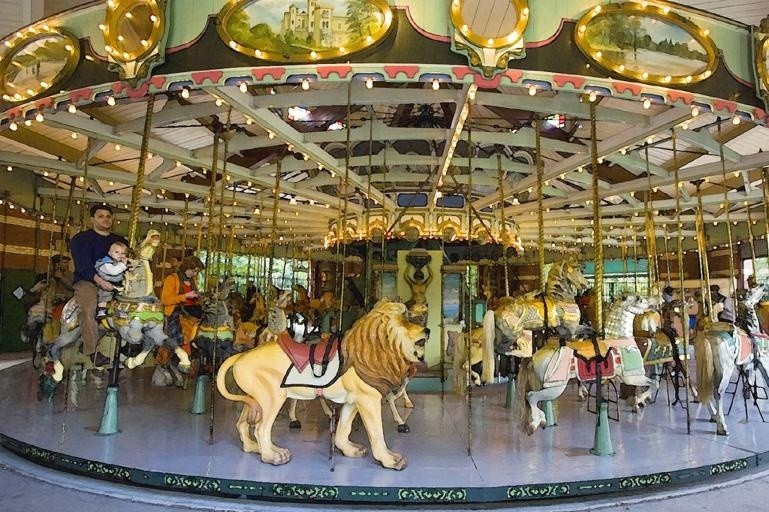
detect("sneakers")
[90,352,109,367]
[95,307,106,316]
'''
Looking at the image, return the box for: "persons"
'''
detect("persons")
[18,254,71,344]
[405,262,434,361]
[247,280,259,300]
[663,286,674,303]
[93,241,130,319]
[71,205,129,367]
[160,254,204,374]
[710,283,725,304]
[170,256,181,273]
